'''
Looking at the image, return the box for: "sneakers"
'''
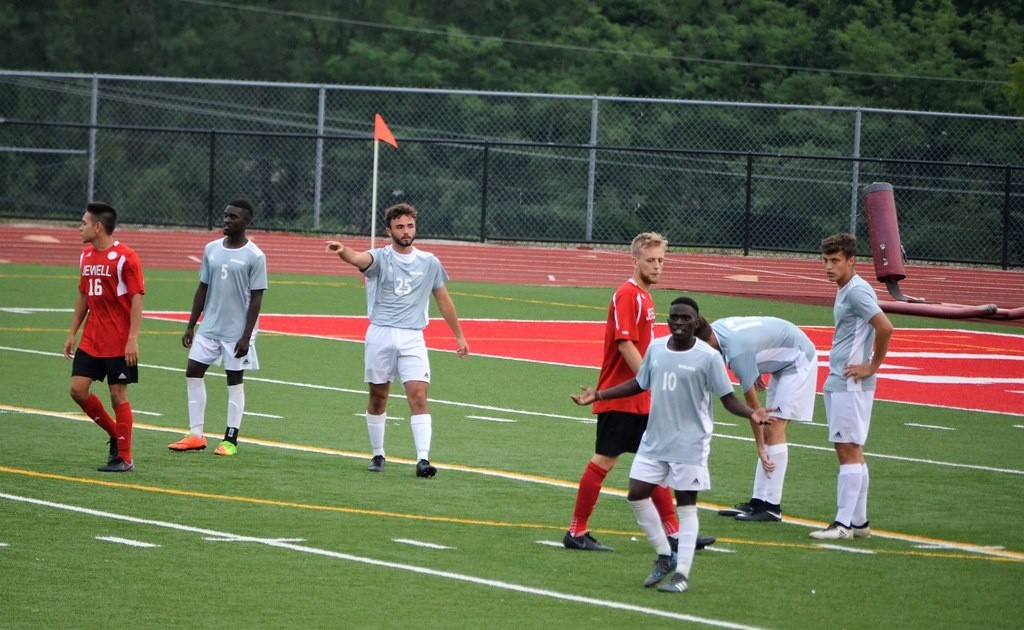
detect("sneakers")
[658,573,688,593]
[735,502,782,521]
[214,441,237,455]
[367,455,385,471]
[416,460,437,478]
[668,536,715,551]
[718,498,762,517]
[808,523,853,540]
[563,531,613,552]
[852,522,870,539]
[168,434,207,452]
[106,438,118,462]
[644,553,677,588]
[97,458,134,472]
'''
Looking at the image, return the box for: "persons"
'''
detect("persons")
[690,314,818,524]
[563,232,716,553]
[326,204,469,480]
[566,297,781,593]
[64,203,146,471]
[810,234,893,541]
[165,199,265,458]
